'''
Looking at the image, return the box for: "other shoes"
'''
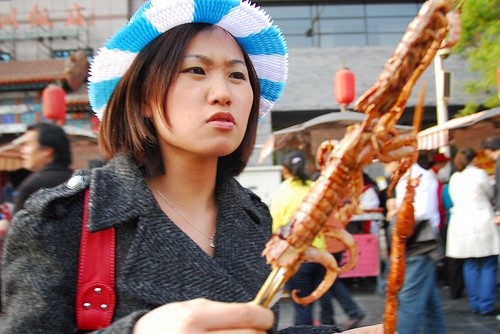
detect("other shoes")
[479,308,500,316]
[342,312,366,330]
[453,293,467,299]
[474,312,479,314]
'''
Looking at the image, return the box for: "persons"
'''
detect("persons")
[0,122,111,246]
[0,1,399,334]
[256,133,500,334]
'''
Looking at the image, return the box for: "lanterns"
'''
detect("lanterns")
[334,69,355,113]
[41,86,66,119]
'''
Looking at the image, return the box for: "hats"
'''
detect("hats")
[85,0,290,128]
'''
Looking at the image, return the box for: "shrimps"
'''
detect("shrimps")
[262,0,464,304]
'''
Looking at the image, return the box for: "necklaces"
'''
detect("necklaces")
[152,183,219,248]
